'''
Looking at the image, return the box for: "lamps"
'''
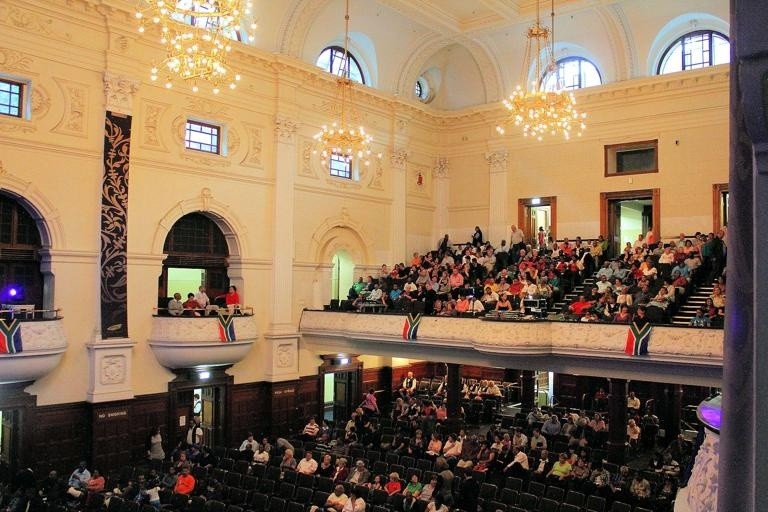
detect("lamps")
[313,0,383,165]
[496,1,586,141]
[131,0,263,95]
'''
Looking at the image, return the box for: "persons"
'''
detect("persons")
[345,389,380,440]
[581,225,727,326]
[349,225,609,320]
[4,391,214,510]
[193,286,210,307]
[240,417,528,512]
[223,284,240,313]
[391,371,501,428]
[502,388,680,507]
[183,292,208,317]
[168,293,185,317]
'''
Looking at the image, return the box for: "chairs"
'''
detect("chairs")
[88,375,678,512]
